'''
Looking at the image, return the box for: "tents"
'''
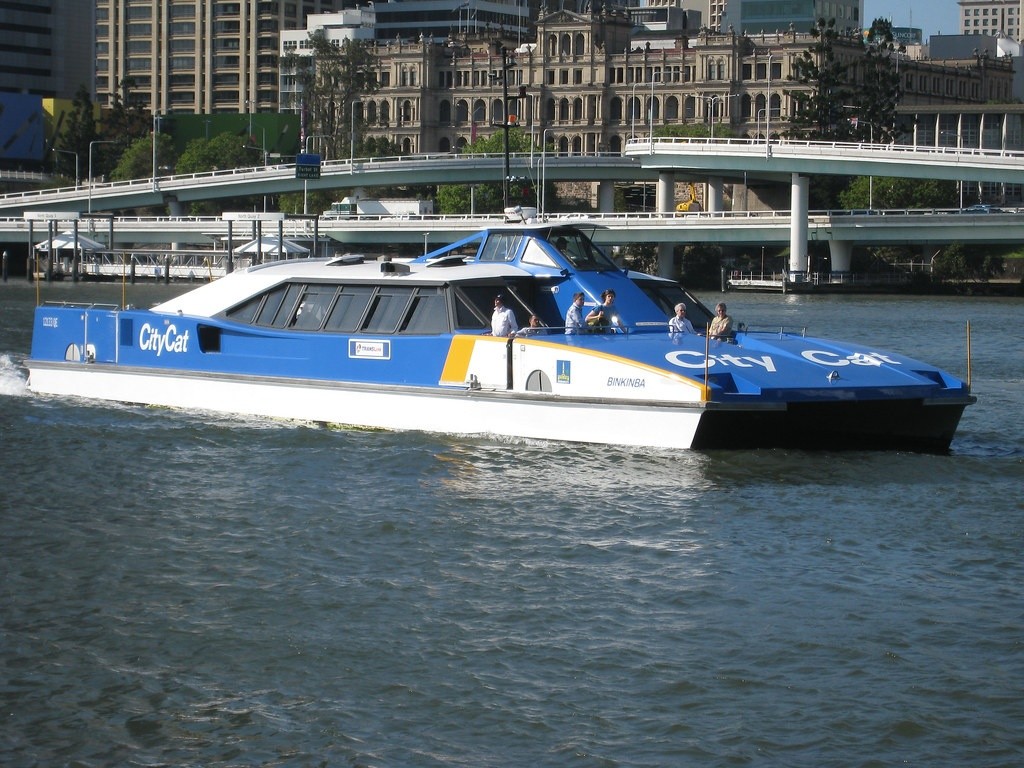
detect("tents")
[33,231,105,263]
[232,233,310,265]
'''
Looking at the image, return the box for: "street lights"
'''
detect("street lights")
[767,54,793,158]
[536,127,569,223]
[632,83,647,141]
[246,99,256,137]
[89,141,115,214]
[152,107,173,195]
[940,131,963,211]
[350,101,368,176]
[650,71,688,154]
[202,120,211,142]
[757,107,787,145]
[51,148,79,192]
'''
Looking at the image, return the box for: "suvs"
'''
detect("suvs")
[954,204,1001,214]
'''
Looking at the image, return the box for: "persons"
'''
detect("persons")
[564,292,590,334]
[586,289,627,333]
[515,314,546,338]
[709,303,732,340]
[669,303,698,334]
[484,294,518,337]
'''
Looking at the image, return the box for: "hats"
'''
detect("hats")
[495,294,505,302]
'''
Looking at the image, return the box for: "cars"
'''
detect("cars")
[561,213,596,220]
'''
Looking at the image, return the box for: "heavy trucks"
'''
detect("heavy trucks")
[331,197,434,220]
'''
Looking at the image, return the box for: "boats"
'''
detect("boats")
[503,206,537,222]
[18,220,980,453]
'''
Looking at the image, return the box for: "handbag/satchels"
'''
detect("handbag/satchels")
[588,318,608,333]
